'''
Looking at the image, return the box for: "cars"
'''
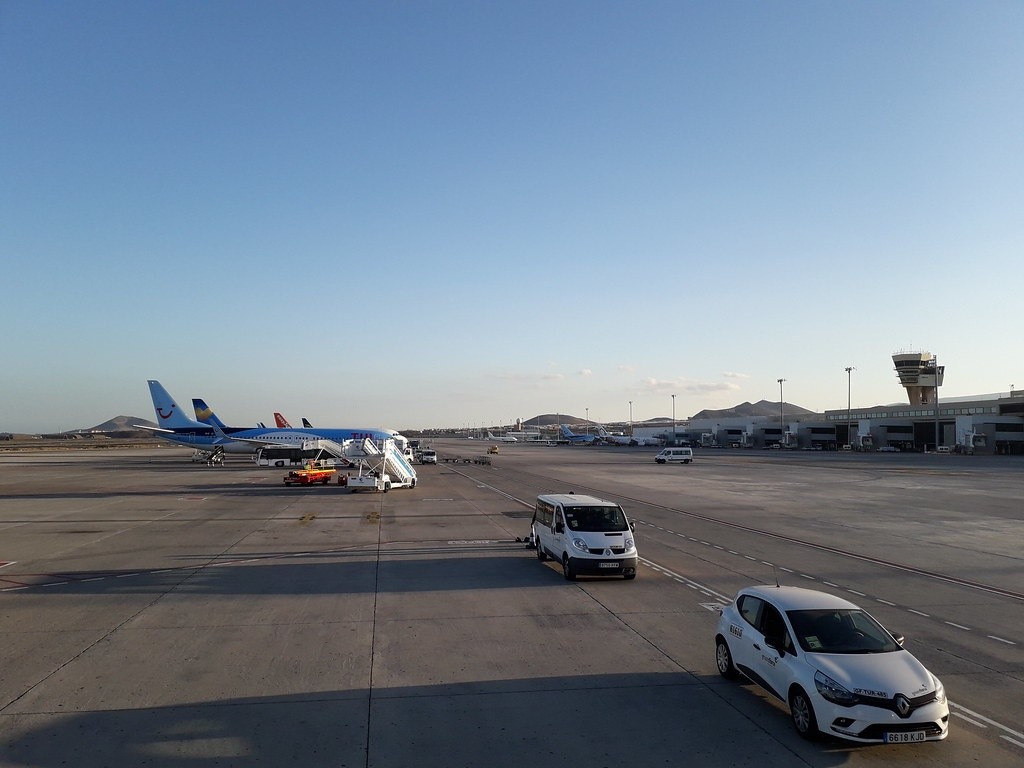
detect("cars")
[715,584,949,745]
[487,445,499,454]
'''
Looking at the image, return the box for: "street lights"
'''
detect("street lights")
[468,418,512,438]
[585,408,589,435]
[629,400,633,432]
[845,366,857,445]
[671,394,677,441]
[557,412,559,440]
[777,377,786,440]
[537,415,539,440]
[521,418,524,439]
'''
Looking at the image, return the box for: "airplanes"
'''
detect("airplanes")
[131,380,408,467]
[487,431,517,444]
[597,424,661,447]
[561,424,607,446]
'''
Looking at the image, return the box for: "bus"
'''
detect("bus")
[255,444,337,467]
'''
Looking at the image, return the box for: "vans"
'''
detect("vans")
[535,494,637,580]
[421,448,437,465]
[654,448,693,464]
